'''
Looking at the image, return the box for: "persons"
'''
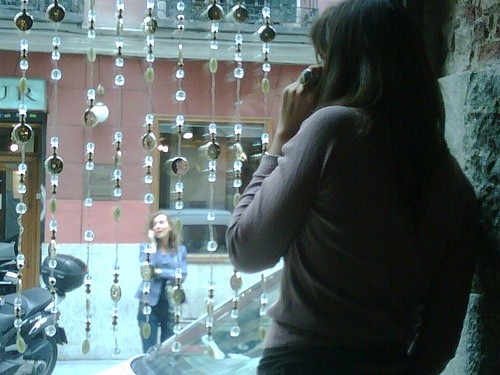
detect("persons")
[223,0,482,375]
[134,210,188,354]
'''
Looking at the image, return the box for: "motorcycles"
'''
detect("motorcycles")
[0,254,87,375]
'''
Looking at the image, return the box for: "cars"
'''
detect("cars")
[92,267,285,375]
[159,209,231,254]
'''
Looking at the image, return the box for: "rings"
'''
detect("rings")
[302,69,312,84]
